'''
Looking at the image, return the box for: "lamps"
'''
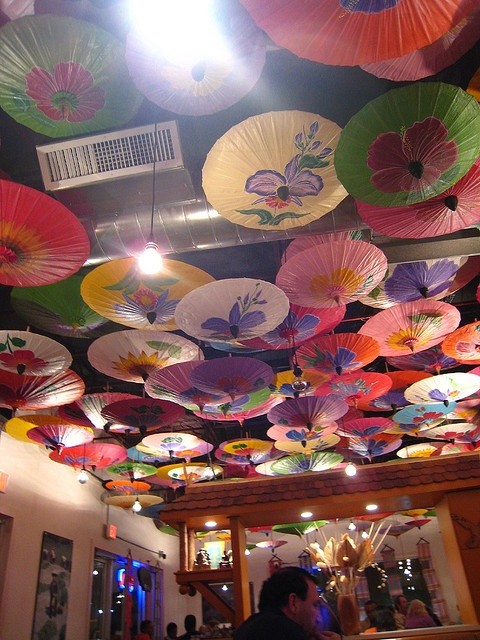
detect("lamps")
[289,312,307,392]
[133,478,142,511]
[78,442,88,484]
[137,122,163,274]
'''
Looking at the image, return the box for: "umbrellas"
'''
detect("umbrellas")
[441,322,480,366]
[352,165,479,239]
[201,109,349,231]
[196,520,330,553]
[126,16,267,119]
[217,432,268,480]
[103,476,161,514]
[9,274,108,338]
[358,16,480,83]
[97,399,182,434]
[290,333,380,375]
[276,440,339,455]
[2,15,143,139]
[59,444,129,472]
[143,361,246,412]
[271,452,343,474]
[80,257,216,333]
[339,416,400,465]
[334,82,480,208]
[75,394,158,433]
[128,431,223,495]
[247,306,346,350]
[174,279,289,342]
[361,254,469,312]
[266,392,348,433]
[26,423,95,455]
[276,243,389,308]
[1,371,85,413]
[266,422,340,440]
[1,330,72,376]
[348,504,439,537]
[281,234,362,263]
[357,298,461,359]
[194,395,287,421]
[0,180,91,286]
[87,328,205,385]
[309,375,393,410]
[248,3,455,68]
[391,373,480,461]
[191,355,276,403]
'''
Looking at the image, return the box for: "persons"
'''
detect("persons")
[177,615,203,639]
[136,620,155,639]
[357,600,381,631]
[365,606,396,634]
[164,622,178,640]
[426,606,443,626]
[404,599,435,627]
[234,565,342,640]
[394,595,410,629]
